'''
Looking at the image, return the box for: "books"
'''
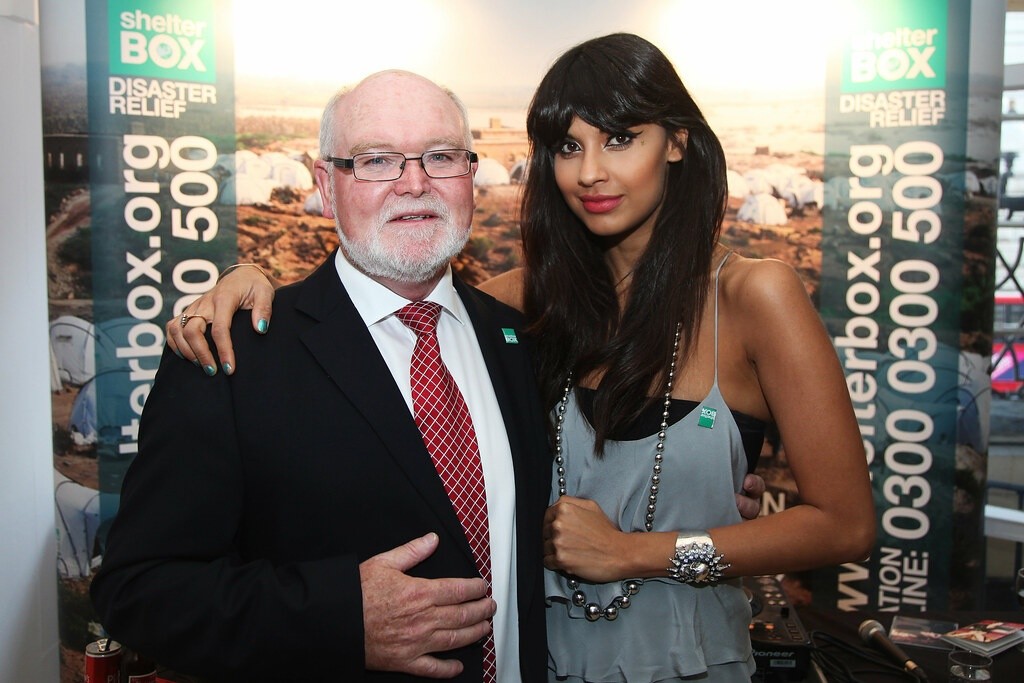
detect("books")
[888,615,958,651]
[939,619,1024,659]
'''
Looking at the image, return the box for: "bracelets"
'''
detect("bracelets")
[216,263,271,286]
[665,528,732,588]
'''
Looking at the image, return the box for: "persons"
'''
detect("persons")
[89,71,765,683]
[166,33,876,683]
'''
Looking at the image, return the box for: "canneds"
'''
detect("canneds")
[85,638,123,683]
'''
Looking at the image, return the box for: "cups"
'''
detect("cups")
[947,650,993,683]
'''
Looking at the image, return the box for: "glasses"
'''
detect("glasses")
[327,147,478,182]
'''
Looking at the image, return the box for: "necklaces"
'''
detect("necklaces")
[556,322,683,622]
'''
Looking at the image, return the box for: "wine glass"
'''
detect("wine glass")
[1015,568,1024,651]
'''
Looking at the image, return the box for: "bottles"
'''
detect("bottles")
[119,643,157,683]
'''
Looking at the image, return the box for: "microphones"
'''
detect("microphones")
[858,620,926,680]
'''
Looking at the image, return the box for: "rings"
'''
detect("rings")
[181,314,207,328]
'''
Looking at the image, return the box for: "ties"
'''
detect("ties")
[394,300,498,683]
